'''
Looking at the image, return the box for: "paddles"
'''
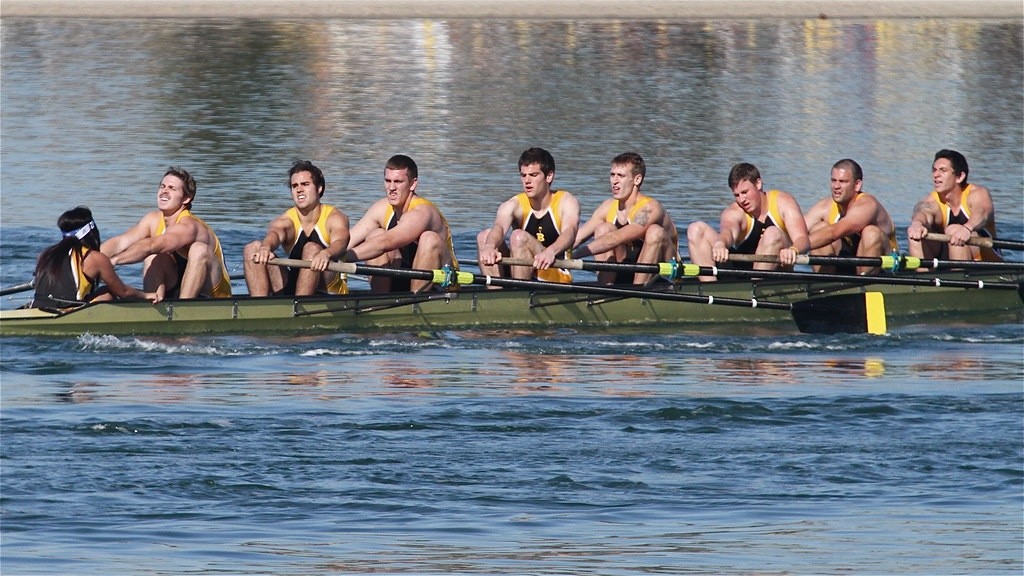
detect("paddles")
[0,281,33,296]
[251,258,888,336]
[456,258,1024,300]
[727,254,1024,275]
[925,234,1024,253]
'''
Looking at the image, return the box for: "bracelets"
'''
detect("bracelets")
[963,226,973,234]
[789,247,799,255]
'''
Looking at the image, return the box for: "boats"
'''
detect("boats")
[1,269,1024,340]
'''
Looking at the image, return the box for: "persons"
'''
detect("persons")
[573,152,681,284]
[243,160,350,297]
[908,149,1003,273]
[34,205,158,308]
[100,170,231,299]
[805,159,898,276]
[341,155,461,292]
[687,163,810,282]
[477,148,580,289]
